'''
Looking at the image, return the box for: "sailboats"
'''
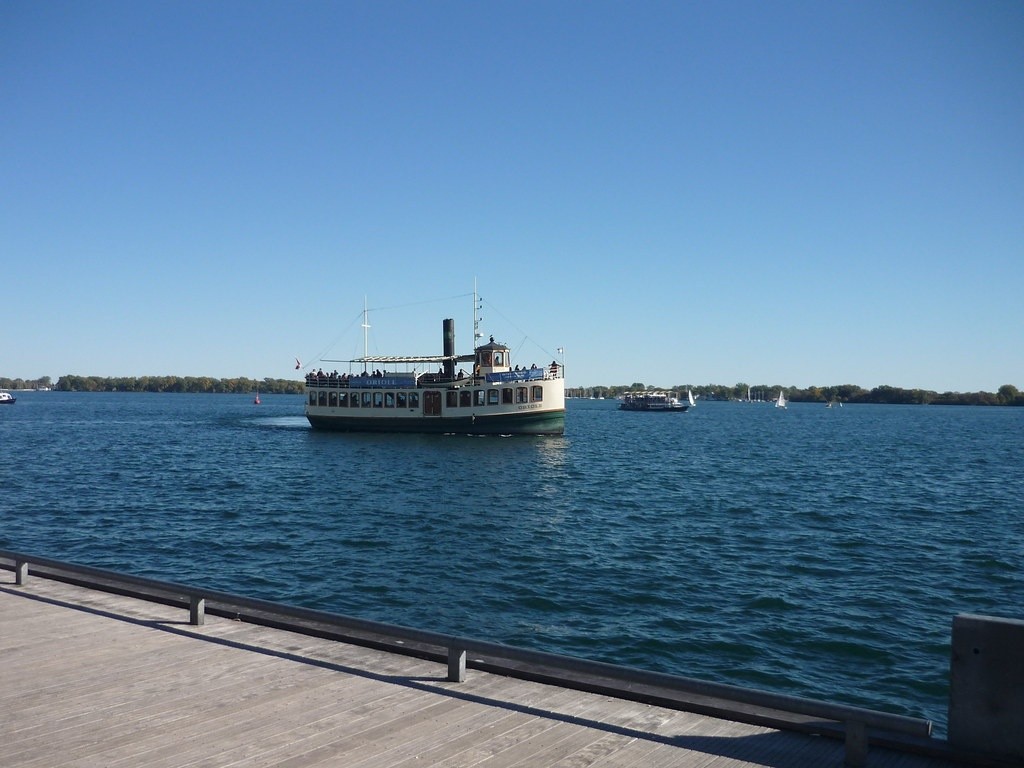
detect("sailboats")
[774,390,788,410]
[688,389,696,408]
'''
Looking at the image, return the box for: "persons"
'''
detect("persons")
[457,368,464,387]
[319,392,383,407]
[494,356,500,366]
[305,368,390,388]
[514,361,561,383]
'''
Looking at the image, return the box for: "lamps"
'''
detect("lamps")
[475,298,482,322]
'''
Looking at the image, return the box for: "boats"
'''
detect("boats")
[303,275,566,435]
[0,388,17,404]
[617,386,690,413]
